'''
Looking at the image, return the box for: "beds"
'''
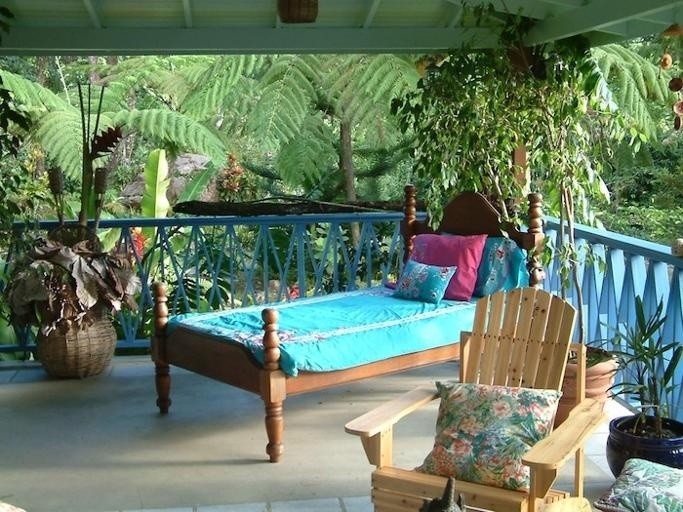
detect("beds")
[149,185,545,463]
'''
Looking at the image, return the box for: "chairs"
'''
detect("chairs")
[346,286,603,511]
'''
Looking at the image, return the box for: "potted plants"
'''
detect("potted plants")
[570,295,683,478]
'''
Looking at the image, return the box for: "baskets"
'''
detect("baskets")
[34,317,117,379]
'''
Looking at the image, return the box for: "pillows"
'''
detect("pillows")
[390,261,457,304]
[441,232,529,299]
[416,380,562,492]
[411,234,488,302]
[593,458,683,512]
